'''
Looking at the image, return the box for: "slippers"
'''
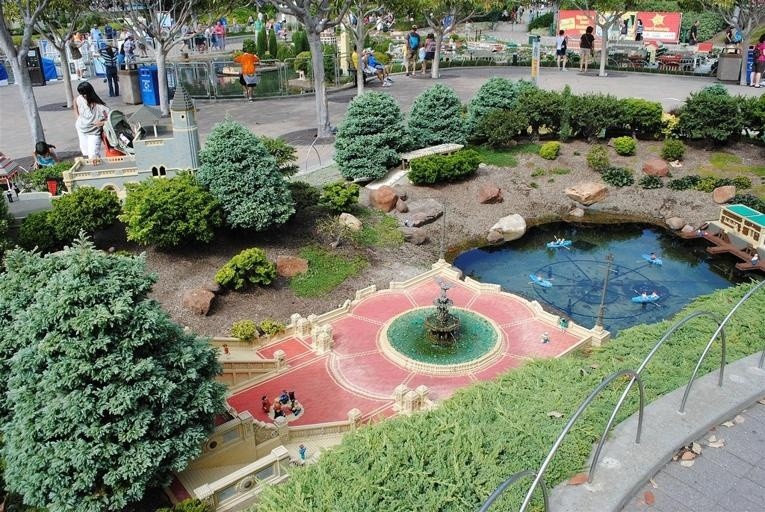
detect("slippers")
[750,83,763,88]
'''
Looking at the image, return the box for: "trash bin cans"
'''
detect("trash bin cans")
[117,61,169,106]
[529,35,537,45]
[0,63,9,87]
[717,47,755,84]
[513,53,517,65]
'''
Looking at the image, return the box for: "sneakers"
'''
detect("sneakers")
[381,77,394,87]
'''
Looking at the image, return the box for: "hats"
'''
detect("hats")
[411,24,418,29]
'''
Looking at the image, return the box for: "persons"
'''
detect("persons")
[650,252,656,260]
[32,142,59,170]
[619,20,627,40]
[560,318,567,334]
[578,26,595,72]
[72,82,109,161]
[512,3,545,24]
[751,253,758,265]
[651,291,657,299]
[262,390,300,419]
[222,344,230,356]
[632,19,643,41]
[556,30,568,71]
[689,21,699,46]
[642,291,646,299]
[554,238,565,245]
[537,274,543,282]
[725,26,735,46]
[749,33,765,88]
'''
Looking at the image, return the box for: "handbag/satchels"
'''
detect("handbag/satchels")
[243,72,257,84]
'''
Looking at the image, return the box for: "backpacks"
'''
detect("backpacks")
[752,44,762,59]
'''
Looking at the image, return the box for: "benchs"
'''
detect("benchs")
[469,50,497,66]
[347,66,368,87]
